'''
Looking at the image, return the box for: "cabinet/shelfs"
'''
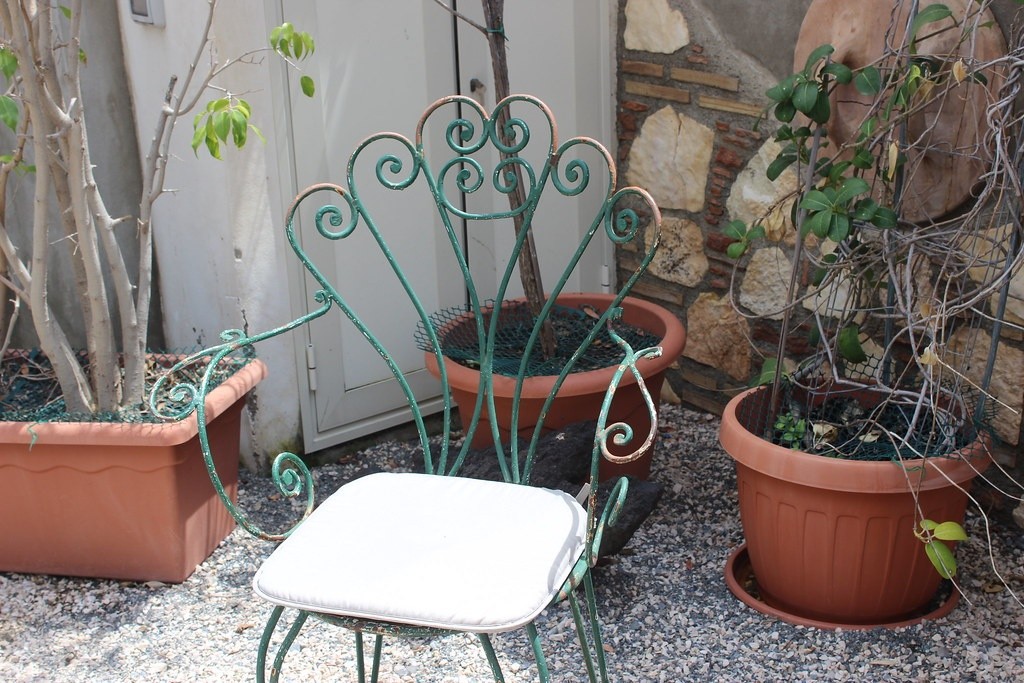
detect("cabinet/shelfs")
[117,0,621,469]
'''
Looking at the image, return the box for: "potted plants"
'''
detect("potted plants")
[425,0,687,486]
[0,0,315,583]
[719,0,1024,630]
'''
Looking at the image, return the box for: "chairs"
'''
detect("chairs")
[148,94,663,683]
